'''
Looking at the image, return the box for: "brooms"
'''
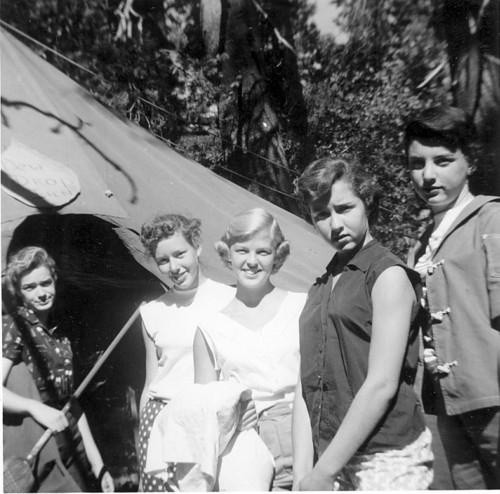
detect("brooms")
[6,300,148,493]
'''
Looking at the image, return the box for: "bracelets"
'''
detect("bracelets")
[97,464,110,492]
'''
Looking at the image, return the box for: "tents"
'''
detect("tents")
[0,14,339,493]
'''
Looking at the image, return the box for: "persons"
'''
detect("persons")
[398,103,500,490]
[285,153,436,491]
[1,246,117,492]
[133,211,238,491]
[194,204,309,491]
